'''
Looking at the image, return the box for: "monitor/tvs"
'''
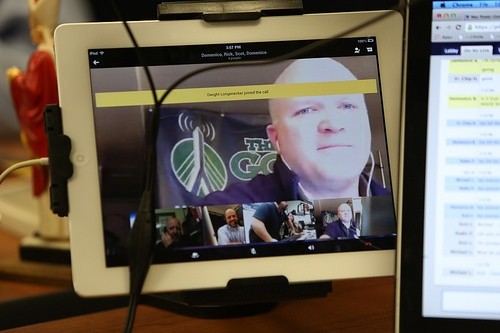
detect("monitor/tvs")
[395,0,500,333]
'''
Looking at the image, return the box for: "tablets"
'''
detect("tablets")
[53,10,404,298]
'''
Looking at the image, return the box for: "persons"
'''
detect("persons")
[315,202,362,243]
[197,55,394,207]
[248,202,290,245]
[283,213,301,240]
[215,208,247,246]
[156,217,200,252]
[179,206,205,250]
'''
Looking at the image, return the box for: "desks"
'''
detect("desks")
[0,144,395,333]
